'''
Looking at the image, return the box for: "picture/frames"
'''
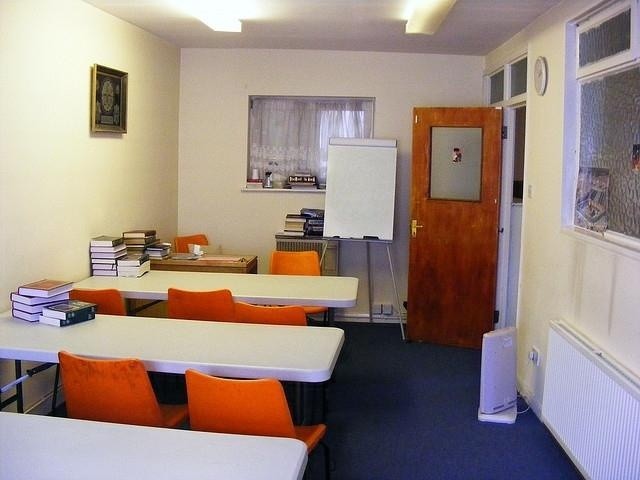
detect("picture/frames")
[90,63,129,134]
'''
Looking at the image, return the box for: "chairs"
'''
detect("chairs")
[70,288,127,317]
[58,353,188,428]
[235,301,308,325]
[175,234,209,253]
[184,368,326,477]
[167,288,236,322]
[269,249,320,276]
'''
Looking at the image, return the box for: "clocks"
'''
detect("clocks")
[533,57,548,97]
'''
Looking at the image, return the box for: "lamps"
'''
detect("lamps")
[405,0,459,37]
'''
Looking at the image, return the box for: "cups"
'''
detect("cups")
[252,169,259,179]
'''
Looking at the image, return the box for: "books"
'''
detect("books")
[245,171,320,191]
[12,309,43,322]
[18,279,73,299]
[12,299,71,314]
[39,313,95,327]
[283,207,325,238]
[43,299,97,321]
[10,291,70,306]
[88,228,172,278]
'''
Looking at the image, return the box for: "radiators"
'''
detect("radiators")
[274,234,338,278]
[537,319,639,480]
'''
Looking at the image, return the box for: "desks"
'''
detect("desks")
[1,308,345,477]
[1,412,308,479]
[146,254,258,274]
[73,270,359,328]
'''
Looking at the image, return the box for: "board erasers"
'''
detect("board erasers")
[363,236,378,240]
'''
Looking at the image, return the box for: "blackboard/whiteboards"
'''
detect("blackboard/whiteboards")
[323,144,397,243]
